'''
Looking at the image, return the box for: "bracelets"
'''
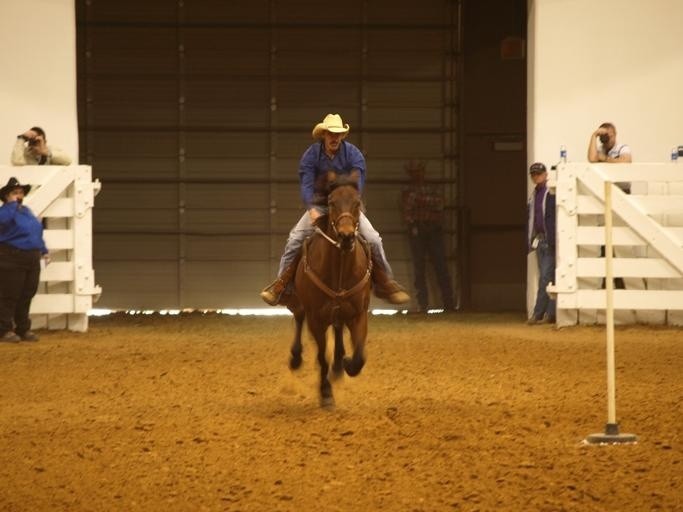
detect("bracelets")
[18,134,28,142]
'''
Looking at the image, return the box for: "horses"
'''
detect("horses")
[287,166,372,410]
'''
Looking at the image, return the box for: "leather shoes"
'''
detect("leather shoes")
[374,289,409,304]
[260,280,284,305]
[526,313,537,323]
[536,312,553,324]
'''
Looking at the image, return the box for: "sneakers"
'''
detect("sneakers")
[20,330,38,340]
[0,331,20,342]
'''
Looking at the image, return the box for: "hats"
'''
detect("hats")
[312,113,350,140]
[530,163,546,174]
[0,178,30,202]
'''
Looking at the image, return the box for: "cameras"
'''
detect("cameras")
[29,139,36,146]
[600,134,609,143]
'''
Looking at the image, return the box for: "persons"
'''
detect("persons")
[396,155,458,315]
[259,114,410,305]
[0,178,53,341]
[10,126,70,167]
[588,123,634,289]
[524,162,555,326]
